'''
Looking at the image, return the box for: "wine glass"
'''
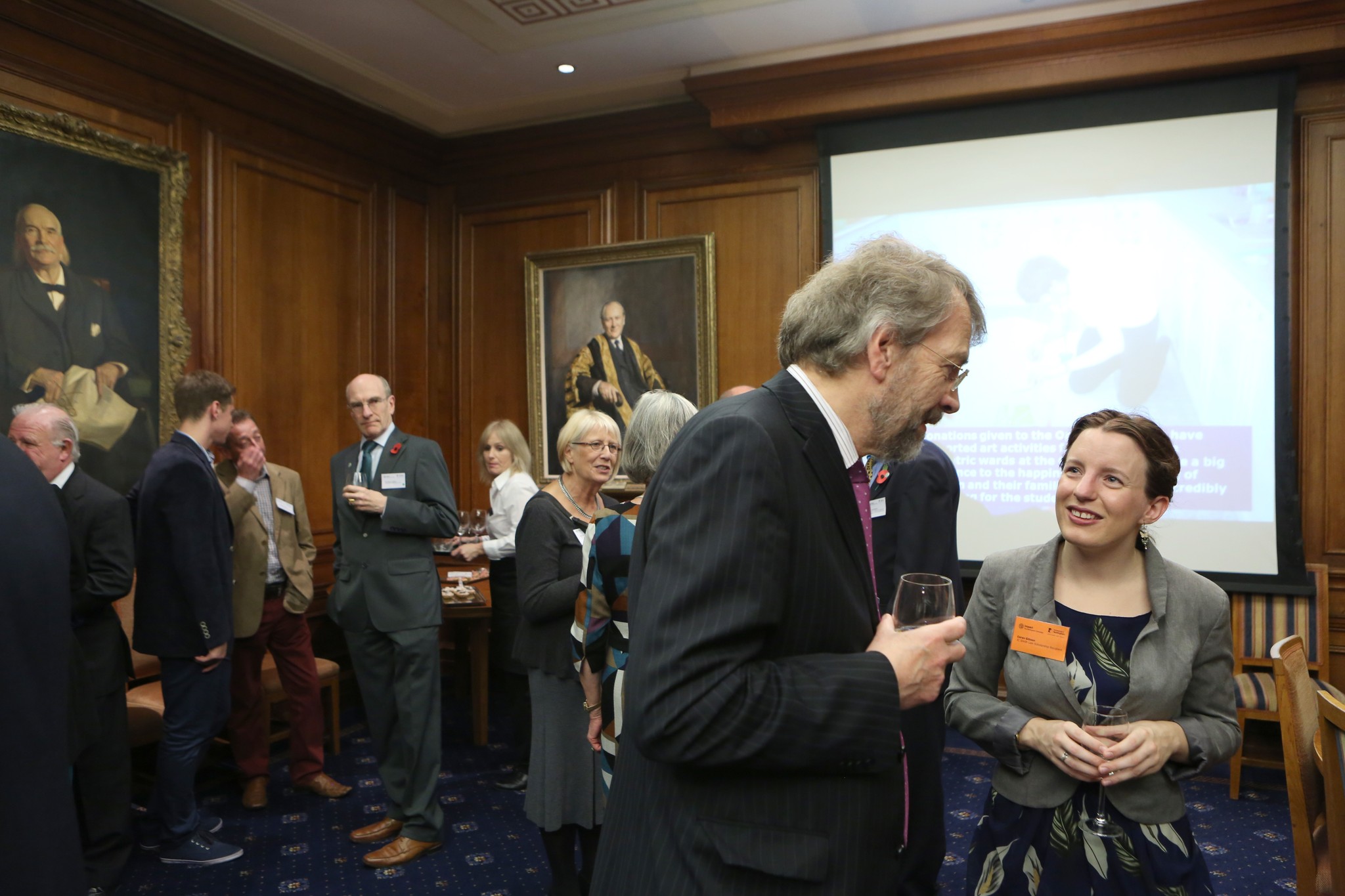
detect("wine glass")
[456,511,470,546]
[470,509,488,543]
[1077,705,1128,838]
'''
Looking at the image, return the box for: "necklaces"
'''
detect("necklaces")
[558,474,600,519]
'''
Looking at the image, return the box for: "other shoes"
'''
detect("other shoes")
[88,886,119,896]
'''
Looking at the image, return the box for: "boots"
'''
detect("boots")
[538,825,602,895]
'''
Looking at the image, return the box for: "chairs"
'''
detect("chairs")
[1226,559,1345,896]
[208,653,343,787]
[91,571,175,817]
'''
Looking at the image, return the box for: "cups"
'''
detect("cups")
[892,573,955,632]
[345,472,367,501]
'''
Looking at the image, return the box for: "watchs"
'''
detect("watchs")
[1015,734,1019,745]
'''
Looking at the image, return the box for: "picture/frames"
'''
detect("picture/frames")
[519,230,720,498]
[0,100,193,495]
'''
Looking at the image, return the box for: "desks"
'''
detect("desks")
[436,556,510,753]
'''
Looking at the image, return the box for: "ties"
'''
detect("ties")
[614,340,620,350]
[847,457,909,849]
[357,440,379,489]
[864,457,876,483]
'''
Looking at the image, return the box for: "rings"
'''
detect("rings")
[1061,752,1068,762]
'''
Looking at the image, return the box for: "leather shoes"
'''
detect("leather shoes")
[494,773,528,790]
[362,836,442,868]
[297,772,352,796]
[351,817,404,842]
[241,776,269,809]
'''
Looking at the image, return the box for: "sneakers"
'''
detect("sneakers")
[140,816,223,851]
[160,834,244,865]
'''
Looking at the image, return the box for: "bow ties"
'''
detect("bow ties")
[41,282,68,296]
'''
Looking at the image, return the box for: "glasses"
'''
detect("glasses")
[348,396,389,414]
[912,339,969,392]
[572,440,623,454]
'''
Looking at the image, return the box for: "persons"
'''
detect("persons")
[584,236,984,896]
[212,408,356,807]
[574,387,700,789]
[446,417,539,787]
[0,203,157,495]
[851,431,960,896]
[940,411,1242,896]
[515,383,606,896]
[0,401,135,896]
[572,300,667,474]
[325,373,460,864]
[720,385,757,400]
[134,369,244,866]
